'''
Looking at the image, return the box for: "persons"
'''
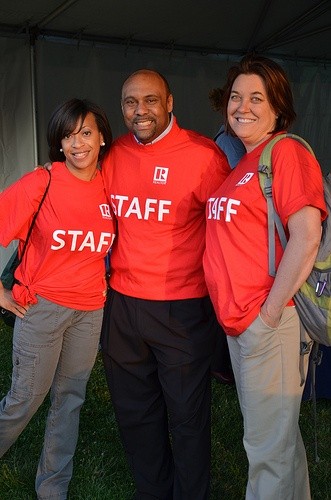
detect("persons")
[0,99,117,500]
[101,71,234,500]
[204,57,329,500]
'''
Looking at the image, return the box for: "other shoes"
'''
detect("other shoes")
[211,370,234,384]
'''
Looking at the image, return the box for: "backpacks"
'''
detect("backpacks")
[0,168,50,327]
[258,133,331,346]
